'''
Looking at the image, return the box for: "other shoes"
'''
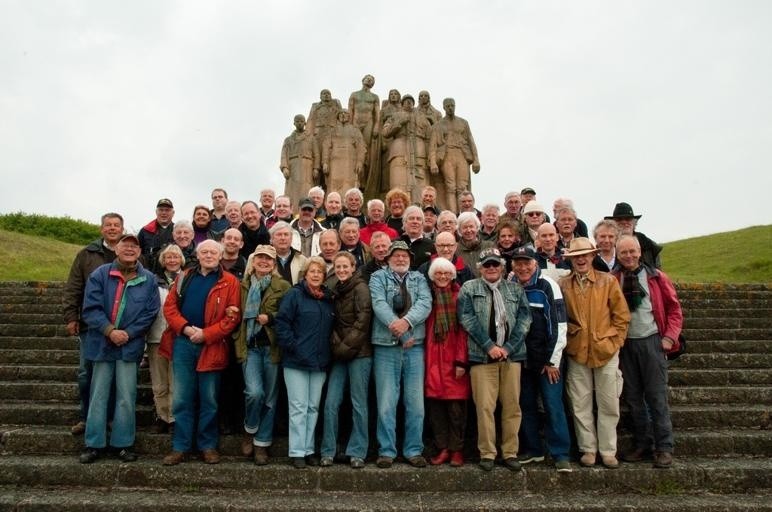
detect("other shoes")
[294,457,307,469]
[626,444,655,463]
[479,458,494,471]
[602,455,619,469]
[501,458,522,472]
[580,452,597,466]
[306,454,320,466]
[320,456,333,467]
[655,451,673,468]
[450,450,465,467]
[241,435,254,455]
[407,455,427,468]
[377,456,393,469]
[518,452,545,464]
[555,461,572,472]
[429,448,450,465]
[254,446,270,465]
[350,456,364,468]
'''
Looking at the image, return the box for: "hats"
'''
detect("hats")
[119,234,139,245]
[522,187,544,214]
[604,202,642,220]
[254,244,276,259]
[157,199,173,208]
[384,240,415,265]
[298,197,315,208]
[560,237,598,257]
[480,247,534,266]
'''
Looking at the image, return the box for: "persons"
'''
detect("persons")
[593,203,662,271]
[62,213,124,435]
[608,235,683,466]
[137,186,590,471]
[79,234,161,463]
[558,237,630,466]
[279,75,480,218]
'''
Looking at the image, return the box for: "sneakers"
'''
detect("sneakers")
[155,420,221,465]
[72,422,137,463]
